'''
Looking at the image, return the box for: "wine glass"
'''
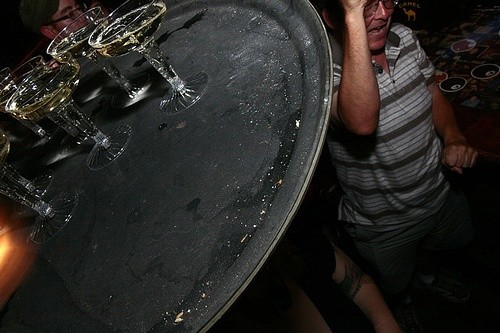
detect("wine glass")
[88,0,209,113]
[0,67,60,148]
[1,180,79,243]
[5,52,131,170]
[46,6,150,107]
[0,128,52,216]
[0,55,97,154]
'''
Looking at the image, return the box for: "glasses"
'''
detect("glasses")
[43,2,88,27]
[363,0,398,17]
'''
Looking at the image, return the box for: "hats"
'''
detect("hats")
[19,0,58,32]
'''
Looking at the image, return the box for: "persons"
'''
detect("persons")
[275,0,480,333]
[18,0,112,42]
